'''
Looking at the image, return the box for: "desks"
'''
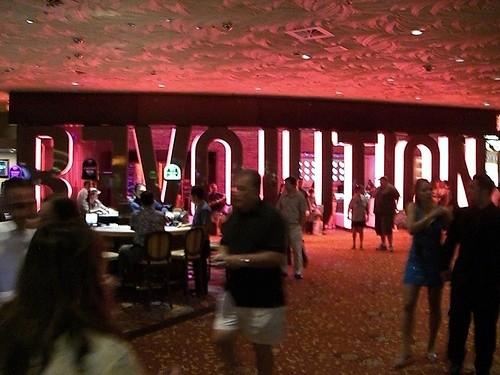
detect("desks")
[90,225,191,237]
[98,214,131,225]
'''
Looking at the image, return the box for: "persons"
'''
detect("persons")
[189,185,212,297]
[277,178,323,269]
[372,176,400,252]
[348,183,370,249]
[207,183,227,236]
[390,178,456,371]
[434,172,500,375]
[275,177,306,279]
[365,180,378,198]
[431,178,450,209]
[117,182,228,298]
[209,167,290,375]
[0,176,149,375]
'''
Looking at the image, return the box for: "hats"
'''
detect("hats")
[88,188,101,194]
[378,176,388,180]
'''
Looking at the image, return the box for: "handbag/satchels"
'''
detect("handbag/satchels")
[312,220,323,236]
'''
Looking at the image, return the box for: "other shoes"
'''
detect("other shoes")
[395,355,417,369]
[295,273,303,279]
[388,246,393,251]
[427,351,437,361]
[376,244,387,250]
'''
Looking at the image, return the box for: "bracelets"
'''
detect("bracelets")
[240,254,252,266]
[424,216,429,222]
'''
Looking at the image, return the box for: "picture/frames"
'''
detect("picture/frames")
[0,159,10,178]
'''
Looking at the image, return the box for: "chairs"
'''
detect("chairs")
[121,230,173,309]
[100,252,119,285]
[171,227,205,304]
